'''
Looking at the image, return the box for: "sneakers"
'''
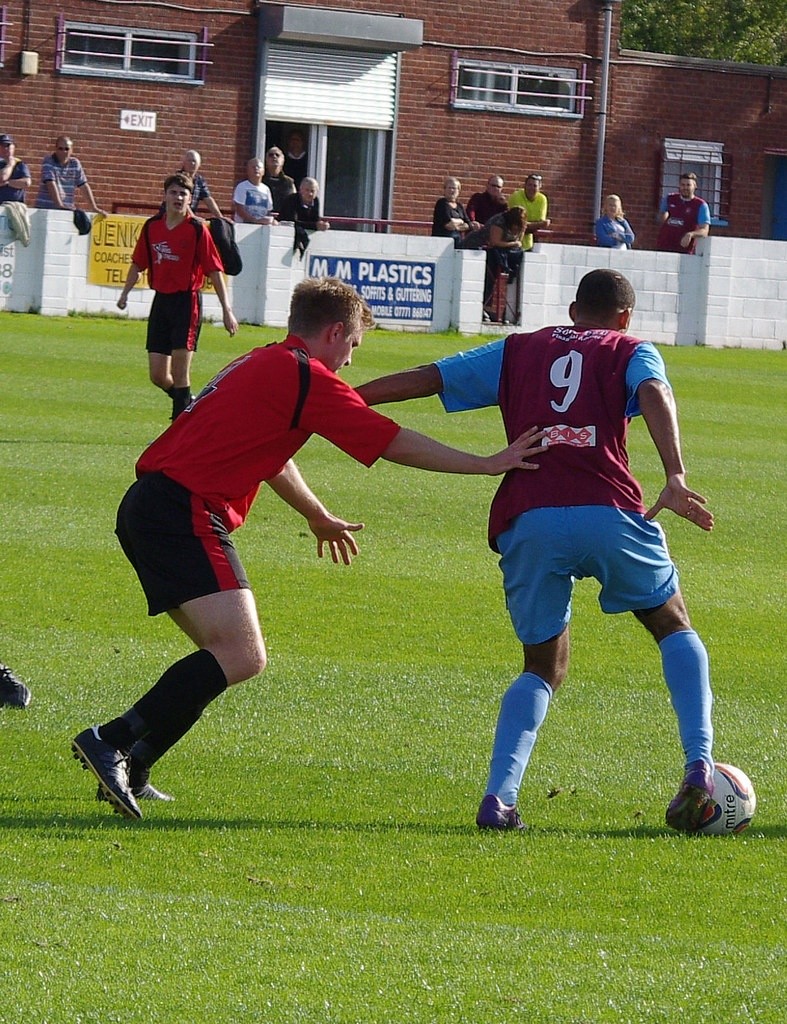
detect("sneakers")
[665,760,715,834]
[476,794,526,830]
[71,724,143,819]
[0,663,32,707]
[95,782,176,803]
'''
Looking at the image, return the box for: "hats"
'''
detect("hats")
[74,209,91,235]
[0,134,13,144]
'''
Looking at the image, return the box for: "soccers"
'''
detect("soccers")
[696,762,756,835]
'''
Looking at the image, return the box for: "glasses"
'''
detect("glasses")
[56,146,72,151]
[530,174,543,180]
[489,183,502,188]
[268,153,283,158]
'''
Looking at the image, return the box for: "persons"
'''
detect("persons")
[34,135,109,217]
[655,171,711,255]
[458,203,528,321]
[284,129,308,190]
[232,156,273,224]
[180,149,236,224]
[352,269,715,834]
[431,176,472,250]
[508,172,551,252]
[116,173,239,425]
[264,146,297,210]
[277,176,331,230]
[466,175,508,230]
[0,134,32,205]
[327,138,359,213]
[594,195,636,250]
[71,274,547,823]
